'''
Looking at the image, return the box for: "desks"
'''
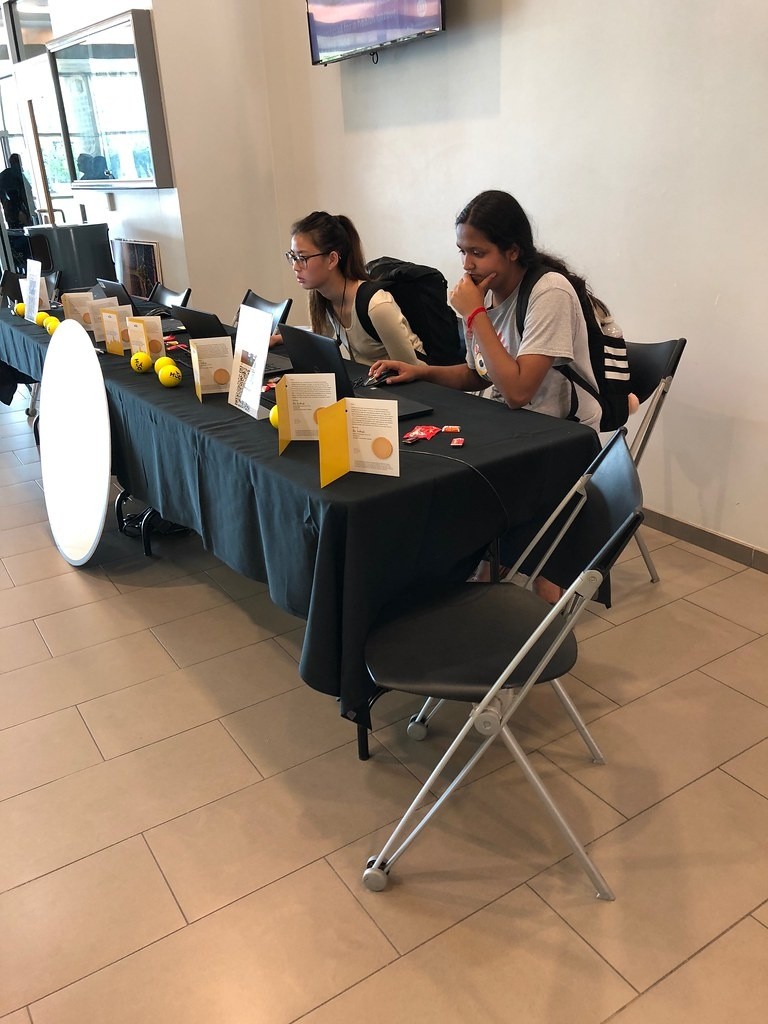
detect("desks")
[0,295,611,759]
[23,223,117,291]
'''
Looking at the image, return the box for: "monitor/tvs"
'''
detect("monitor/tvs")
[307,0,446,66]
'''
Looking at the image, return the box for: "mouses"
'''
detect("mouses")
[146,307,173,318]
[365,370,399,387]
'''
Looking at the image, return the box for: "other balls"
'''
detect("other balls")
[14,303,26,317]
[270,405,281,429]
[154,356,175,374]
[131,352,153,373]
[158,365,183,388]
[34,311,59,335]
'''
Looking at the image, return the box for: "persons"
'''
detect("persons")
[268,211,431,368]
[76,153,115,180]
[368,188,603,614]
[0,153,37,230]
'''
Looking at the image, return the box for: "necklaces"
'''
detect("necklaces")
[331,279,347,345]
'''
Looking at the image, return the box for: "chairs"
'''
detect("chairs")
[455,317,494,397]
[0,269,62,308]
[147,281,192,307]
[363,424,645,904]
[232,289,293,335]
[490,336,687,622]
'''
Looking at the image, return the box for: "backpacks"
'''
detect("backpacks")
[356,256,465,367]
[515,260,630,433]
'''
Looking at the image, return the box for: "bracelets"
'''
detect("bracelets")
[466,306,487,328]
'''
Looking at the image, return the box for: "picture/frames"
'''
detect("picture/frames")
[109,238,162,299]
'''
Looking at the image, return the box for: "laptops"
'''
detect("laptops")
[171,305,294,376]
[277,323,434,421]
[97,278,187,335]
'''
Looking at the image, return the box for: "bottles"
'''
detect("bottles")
[603,316,623,338]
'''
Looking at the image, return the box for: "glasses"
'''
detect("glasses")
[285,250,331,270]
